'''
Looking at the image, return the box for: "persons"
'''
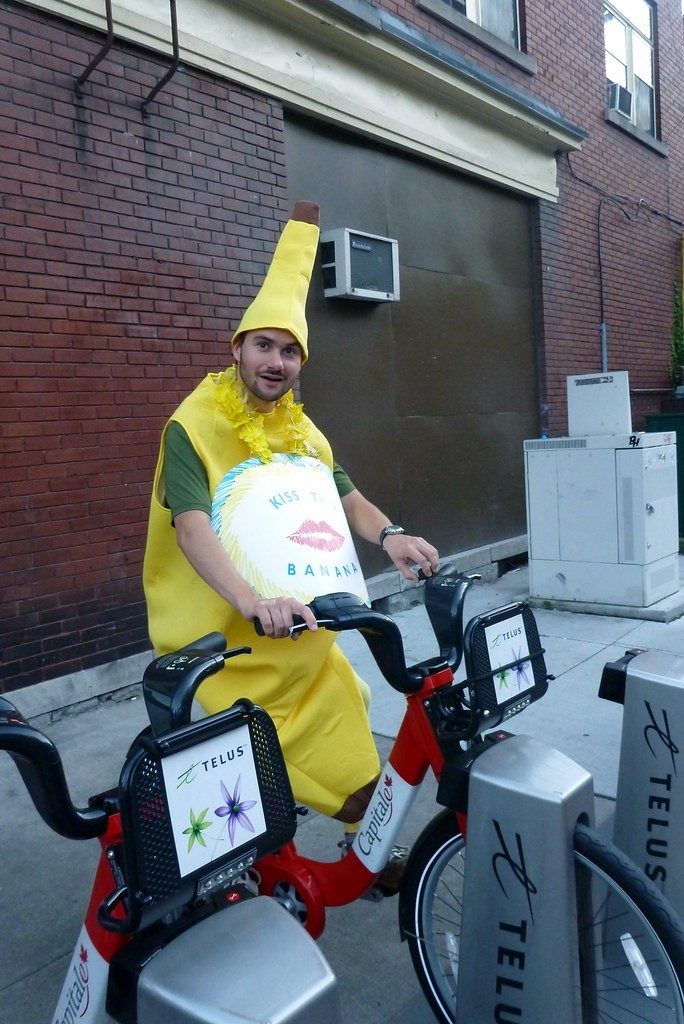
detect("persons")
[144,202,444,894]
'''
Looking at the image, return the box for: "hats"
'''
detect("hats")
[231,201,321,367]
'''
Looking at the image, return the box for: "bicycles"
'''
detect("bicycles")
[0,631,294,1024]
[246,564,683,1023]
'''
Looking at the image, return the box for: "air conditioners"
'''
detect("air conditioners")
[319,227,401,305]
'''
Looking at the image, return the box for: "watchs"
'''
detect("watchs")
[379,525,406,550]
